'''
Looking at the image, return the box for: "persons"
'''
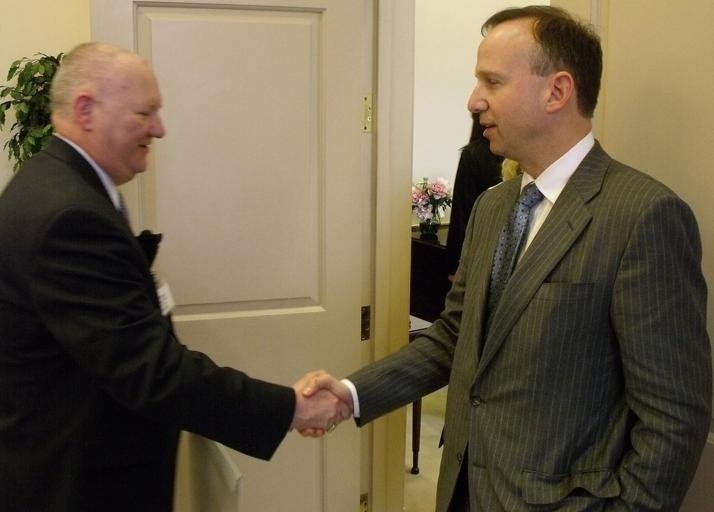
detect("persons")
[439,113,504,298]
[302,4,714,512]
[1,40,353,512]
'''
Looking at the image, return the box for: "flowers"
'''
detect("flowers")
[411,176,454,224]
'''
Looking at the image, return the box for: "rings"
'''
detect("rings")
[327,423,336,434]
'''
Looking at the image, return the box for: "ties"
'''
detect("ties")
[485,184,544,324]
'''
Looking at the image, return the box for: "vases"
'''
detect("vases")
[418,217,441,236]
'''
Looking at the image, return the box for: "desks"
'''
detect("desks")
[412,225,453,324]
